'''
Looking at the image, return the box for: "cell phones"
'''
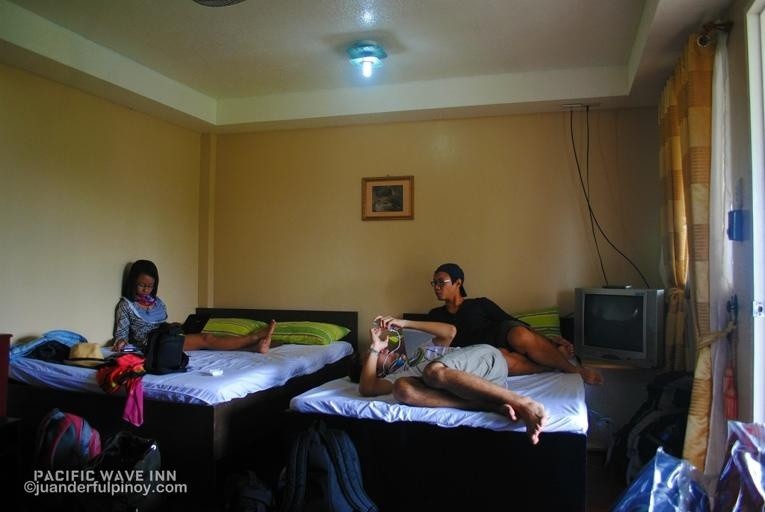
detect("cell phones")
[373,322,400,332]
[121,346,136,353]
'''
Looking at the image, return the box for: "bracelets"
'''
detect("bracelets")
[367,347,380,355]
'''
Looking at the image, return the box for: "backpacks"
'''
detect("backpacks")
[274,421,379,512]
[36,408,101,472]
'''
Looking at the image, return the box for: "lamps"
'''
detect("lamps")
[345,37,386,87]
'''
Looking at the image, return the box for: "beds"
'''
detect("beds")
[287,307,590,511]
[7,306,361,512]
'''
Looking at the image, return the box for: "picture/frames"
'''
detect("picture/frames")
[359,175,415,221]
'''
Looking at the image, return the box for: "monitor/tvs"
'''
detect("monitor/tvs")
[575,288,664,369]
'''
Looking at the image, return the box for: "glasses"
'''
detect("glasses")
[431,279,451,287]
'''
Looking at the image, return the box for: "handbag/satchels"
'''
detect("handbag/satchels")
[79,430,162,483]
[145,322,191,375]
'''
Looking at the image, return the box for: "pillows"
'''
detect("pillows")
[200,314,266,335]
[266,320,355,346]
[508,308,562,344]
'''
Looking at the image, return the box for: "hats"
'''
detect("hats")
[435,264,466,297]
[62,343,104,368]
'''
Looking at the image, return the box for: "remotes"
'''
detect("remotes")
[603,285,632,288]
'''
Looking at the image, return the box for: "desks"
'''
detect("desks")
[582,359,658,462]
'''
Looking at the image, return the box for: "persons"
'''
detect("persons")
[359,315,547,448]
[427,263,602,386]
[112,259,277,355]
